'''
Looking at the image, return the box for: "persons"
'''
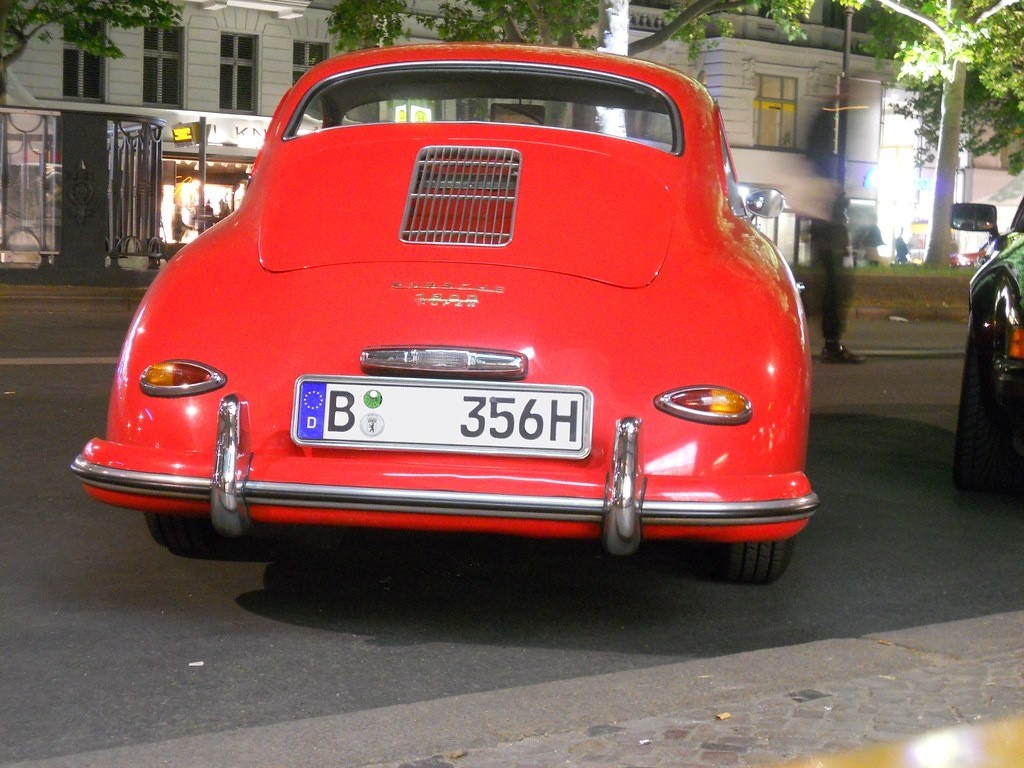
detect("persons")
[168,196,232,242]
[815,218,857,364]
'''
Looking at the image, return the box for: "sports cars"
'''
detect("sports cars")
[69,41,822,584]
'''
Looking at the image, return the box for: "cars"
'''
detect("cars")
[949,189,1024,490]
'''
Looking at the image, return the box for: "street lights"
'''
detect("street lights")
[821,0,865,368]
